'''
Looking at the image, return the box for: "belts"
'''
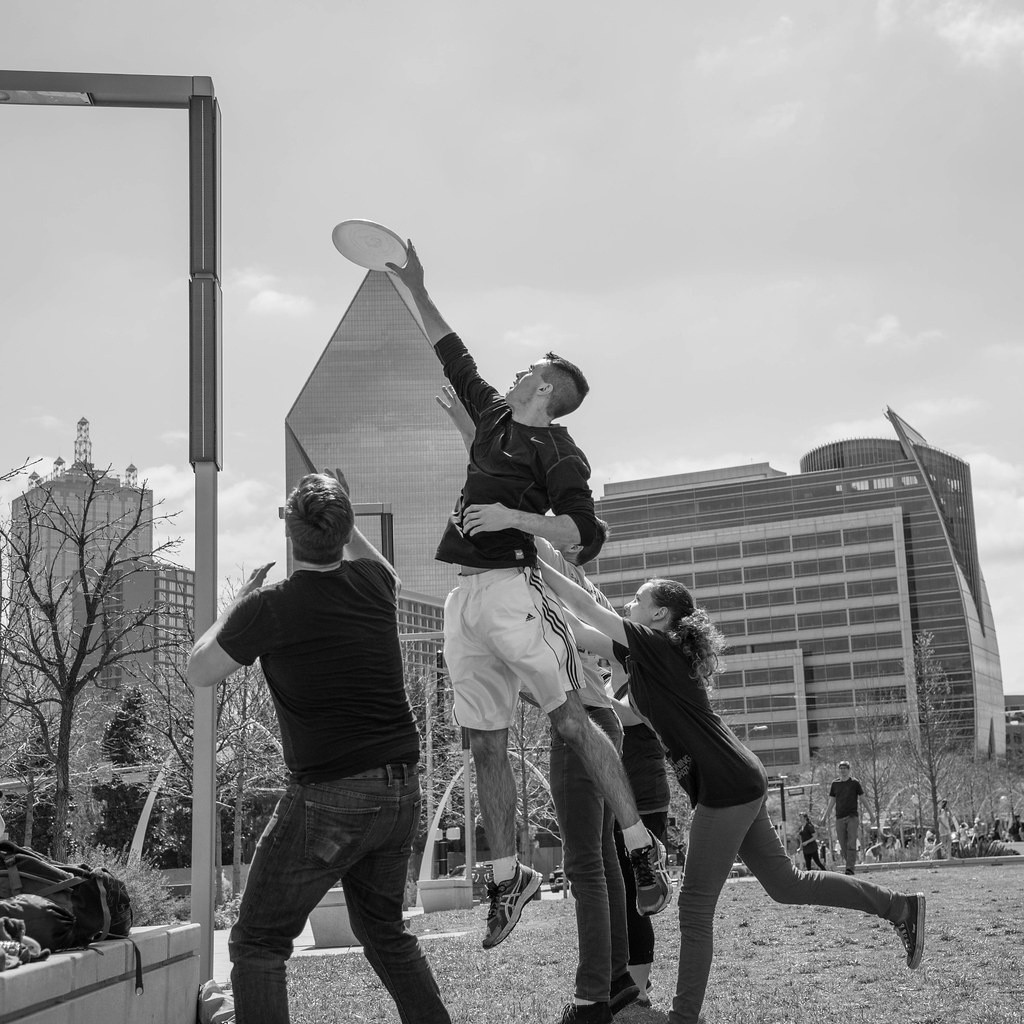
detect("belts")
[344,763,418,779]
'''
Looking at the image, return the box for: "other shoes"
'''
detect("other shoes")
[607,971,641,1018]
[845,870,854,875]
[557,1000,615,1024]
[631,994,652,1010]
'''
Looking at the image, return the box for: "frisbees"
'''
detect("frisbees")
[332,220,408,271]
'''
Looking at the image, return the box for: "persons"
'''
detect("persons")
[387,240,676,947]
[535,527,1024,1024]
[190,475,454,1024]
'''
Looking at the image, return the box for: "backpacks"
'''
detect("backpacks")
[0,839,146,996]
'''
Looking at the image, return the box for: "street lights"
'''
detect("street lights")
[910,794,920,844]
[1000,796,1007,829]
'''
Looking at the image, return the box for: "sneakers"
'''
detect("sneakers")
[623,827,674,916]
[481,862,545,950]
[892,891,927,969]
